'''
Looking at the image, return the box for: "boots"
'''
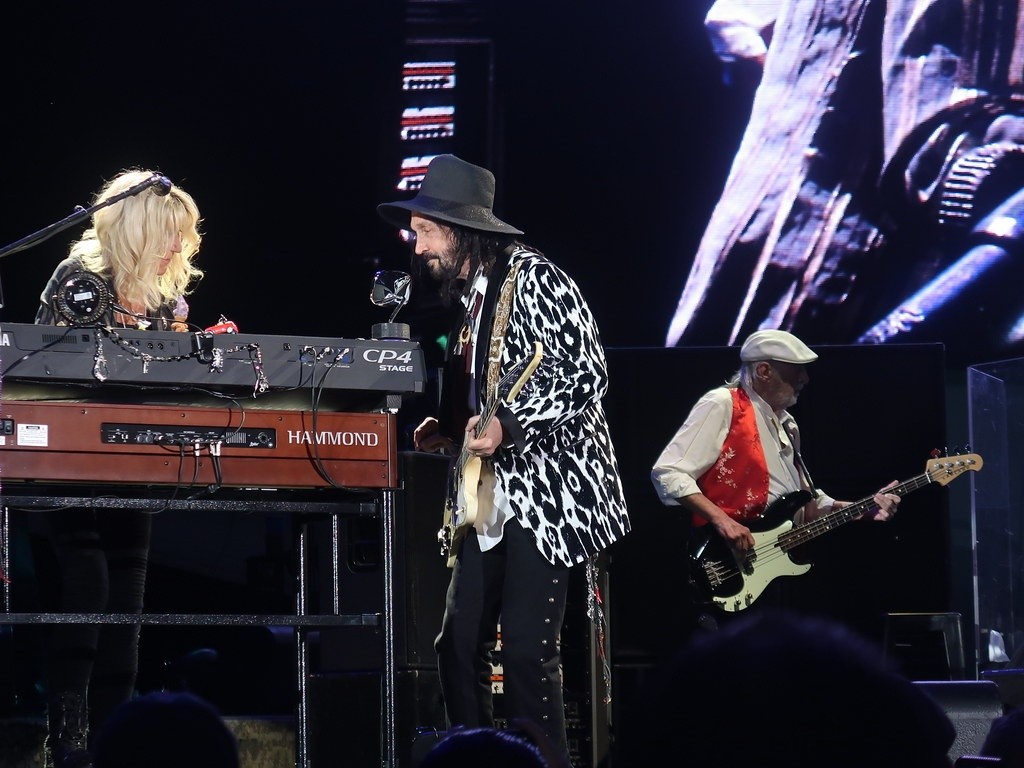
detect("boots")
[41,684,92,768]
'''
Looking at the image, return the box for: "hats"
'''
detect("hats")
[739,329,818,364]
[376,153,525,236]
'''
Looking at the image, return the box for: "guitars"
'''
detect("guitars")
[685,439,985,621]
[436,338,548,577]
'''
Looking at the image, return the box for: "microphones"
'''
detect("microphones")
[151,175,171,197]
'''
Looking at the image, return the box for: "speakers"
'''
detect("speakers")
[307,669,449,768]
[317,452,457,674]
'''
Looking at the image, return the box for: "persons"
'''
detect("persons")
[28,167,205,768]
[651,329,901,632]
[373,154,633,758]
[664,0,1024,356]
[0,610,1023,768]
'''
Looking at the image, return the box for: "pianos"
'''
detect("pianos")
[2,262,437,768]
